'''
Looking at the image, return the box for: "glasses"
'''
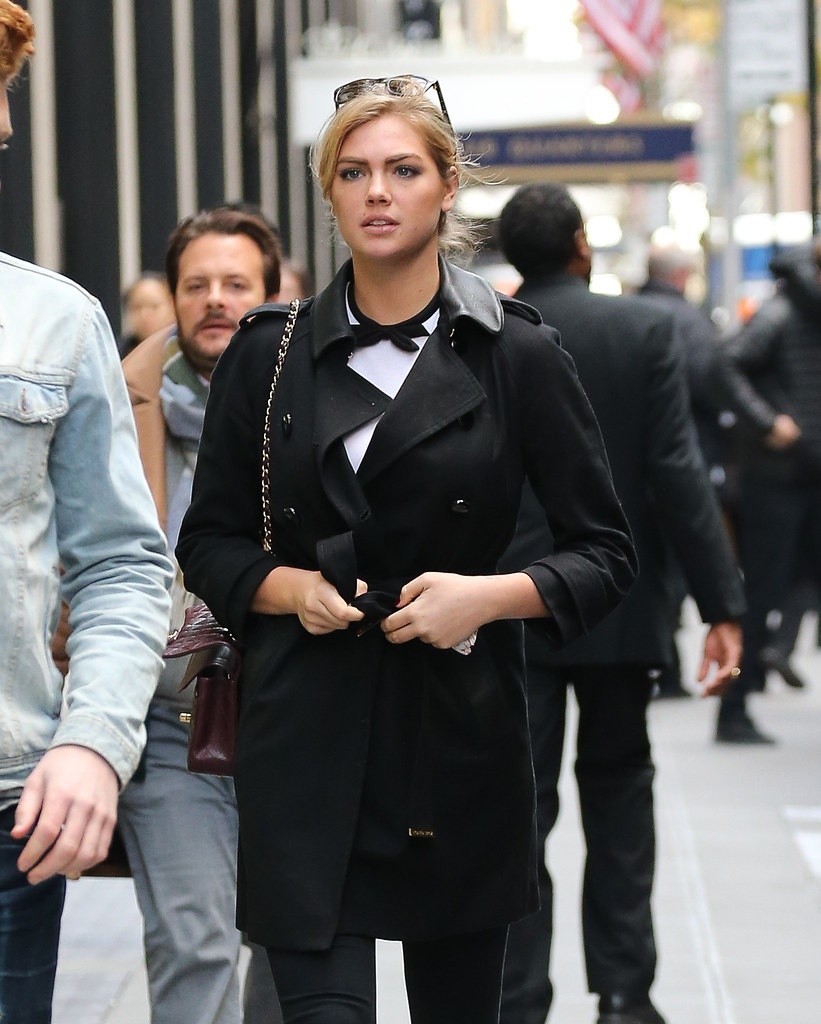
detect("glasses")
[334,74,454,136]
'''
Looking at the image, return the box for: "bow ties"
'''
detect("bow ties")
[348,277,438,351]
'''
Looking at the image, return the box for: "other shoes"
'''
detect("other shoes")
[656,671,691,696]
[758,632,805,687]
[597,990,666,1024]
[716,705,773,744]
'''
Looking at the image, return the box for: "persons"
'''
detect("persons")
[487,182,744,1024]
[0,2,318,1024]
[175,80,636,1024]
[623,232,821,744]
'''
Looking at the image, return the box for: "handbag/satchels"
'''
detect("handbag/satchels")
[162,603,241,776]
